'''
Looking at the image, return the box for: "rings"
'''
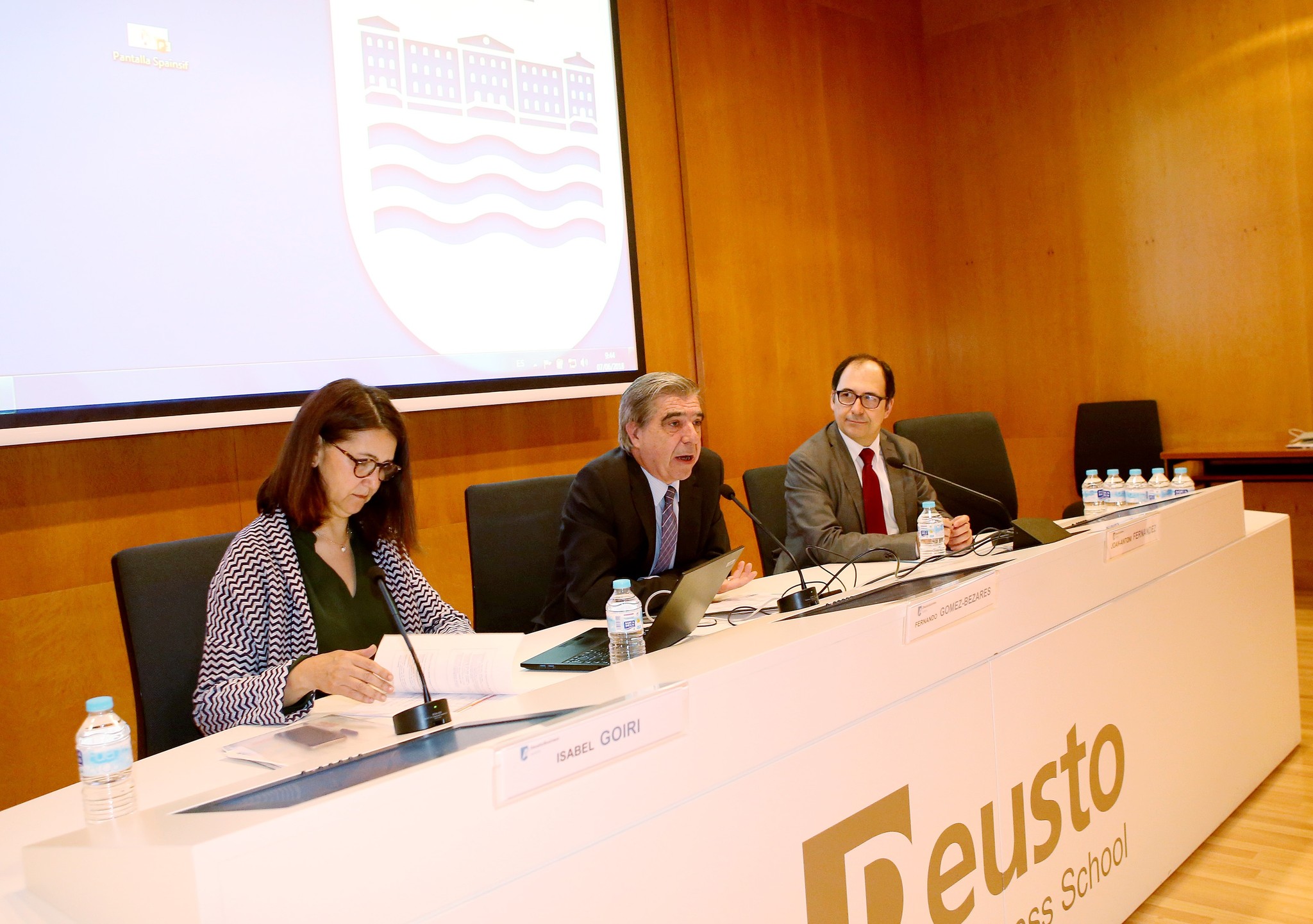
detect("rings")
[726,578,731,581]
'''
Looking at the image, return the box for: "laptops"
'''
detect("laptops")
[519,545,744,670]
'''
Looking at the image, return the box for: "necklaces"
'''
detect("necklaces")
[313,522,350,553]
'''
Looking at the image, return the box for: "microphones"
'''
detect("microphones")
[368,565,452,734]
[718,483,820,613]
[886,456,1014,527]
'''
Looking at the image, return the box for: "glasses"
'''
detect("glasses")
[324,438,402,481]
[832,391,888,408]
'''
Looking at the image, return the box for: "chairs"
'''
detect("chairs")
[743,465,795,584]
[463,472,573,638]
[893,413,1018,538]
[1061,399,1168,528]
[112,533,234,759]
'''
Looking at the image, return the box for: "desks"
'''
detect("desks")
[1160,443,1313,496]
[0,506,1303,923]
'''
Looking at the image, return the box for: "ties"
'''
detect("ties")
[859,448,888,535]
[650,486,677,576]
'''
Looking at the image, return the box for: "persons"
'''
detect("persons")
[532,371,758,633]
[192,379,476,739]
[773,353,973,576]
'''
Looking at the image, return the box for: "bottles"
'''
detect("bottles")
[1169,467,1195,495]
[75,696,140,825]
[605,579,646,665]
[1147,468,1171,501]
[1082,469,1103,519]
[916,501,946,562]
[1101,469,1125,510]
[1124,469,1147,506]
